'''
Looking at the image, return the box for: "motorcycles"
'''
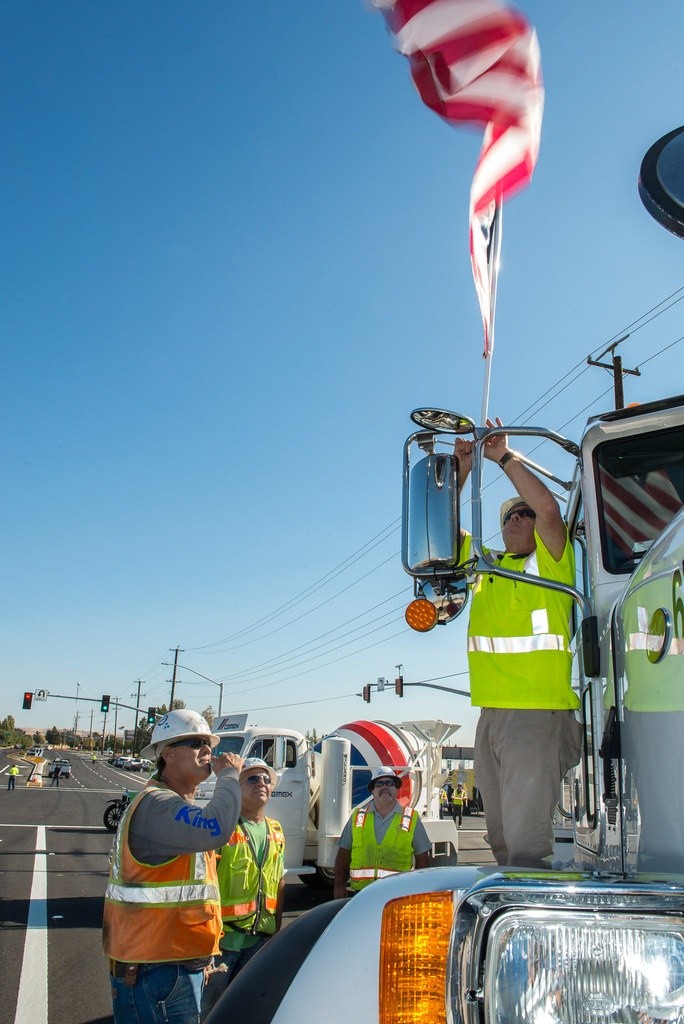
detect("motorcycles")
[103,789,140,832]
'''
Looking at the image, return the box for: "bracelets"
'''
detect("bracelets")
[499,452,516,470]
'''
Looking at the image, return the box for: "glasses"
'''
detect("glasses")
[504,509,536,525]
[168,737,210,749]
[458,785,462,786]
[240,775,272,784]
[373,780,397,787]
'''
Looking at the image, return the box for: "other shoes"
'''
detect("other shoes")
[459,825,462,828]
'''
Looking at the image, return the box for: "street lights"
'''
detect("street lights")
[160,662,224,718]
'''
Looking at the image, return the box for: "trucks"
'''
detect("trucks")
[194,719,462,901]
[198,391,684,1024]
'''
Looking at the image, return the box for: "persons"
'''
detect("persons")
[91,755,96,764]
[451,782,467,827]
[102,709,244,1024]
[50,763,61,787]
[439,788,447,819]
[453,418,582,869]
[334,766,433,900]
[623,568,684,873]
[8,765,19,790]
[446,784,454,816]
[200,757,285,1024]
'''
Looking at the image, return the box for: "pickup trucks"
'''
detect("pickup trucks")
[47,758,72,779]
[107,756,154,773]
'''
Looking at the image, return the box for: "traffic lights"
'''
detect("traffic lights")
[22,692,33,709]
[148,707,156,724]
[101,695,110,712]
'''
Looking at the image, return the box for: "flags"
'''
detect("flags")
[379,0,545,357]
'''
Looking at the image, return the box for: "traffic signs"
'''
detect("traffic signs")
[34,689,49,702]
[377,676,385,691]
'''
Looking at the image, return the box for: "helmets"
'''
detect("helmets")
[368,766,402,792]
[458,781,462,784]
[239,758,277,791]
[140,709,220,759]
[500,497,524,527]
